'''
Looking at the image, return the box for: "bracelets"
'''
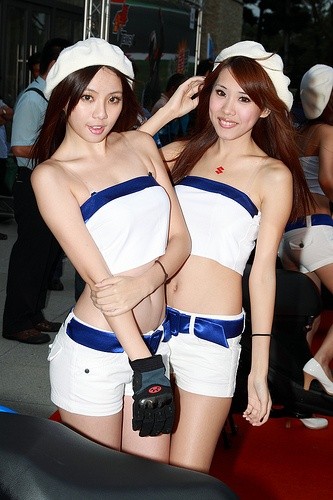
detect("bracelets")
[155,260,168,284]
[250,334,274,337]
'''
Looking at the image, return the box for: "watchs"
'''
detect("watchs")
[2,107,6,116]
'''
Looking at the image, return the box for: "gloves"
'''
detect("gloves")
[129,356,176,439]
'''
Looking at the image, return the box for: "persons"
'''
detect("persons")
[29,37,192,465]
[280,63,333,395]
[150,73,187,115]
[167,57,216,145]
[0,97,13,241]
[26,51,41,78]
[164,40,294,472]
[0,37,72,346]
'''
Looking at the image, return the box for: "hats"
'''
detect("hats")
[44,38,133,103]
[302,64,333,119]
[213,40,293,111]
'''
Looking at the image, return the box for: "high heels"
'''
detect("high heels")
[302,358,333,396]
[285,414,328,429]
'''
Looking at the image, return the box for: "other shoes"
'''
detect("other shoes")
[35,319,61,332]
[2,329,51,344]
[0,233,9,240]
